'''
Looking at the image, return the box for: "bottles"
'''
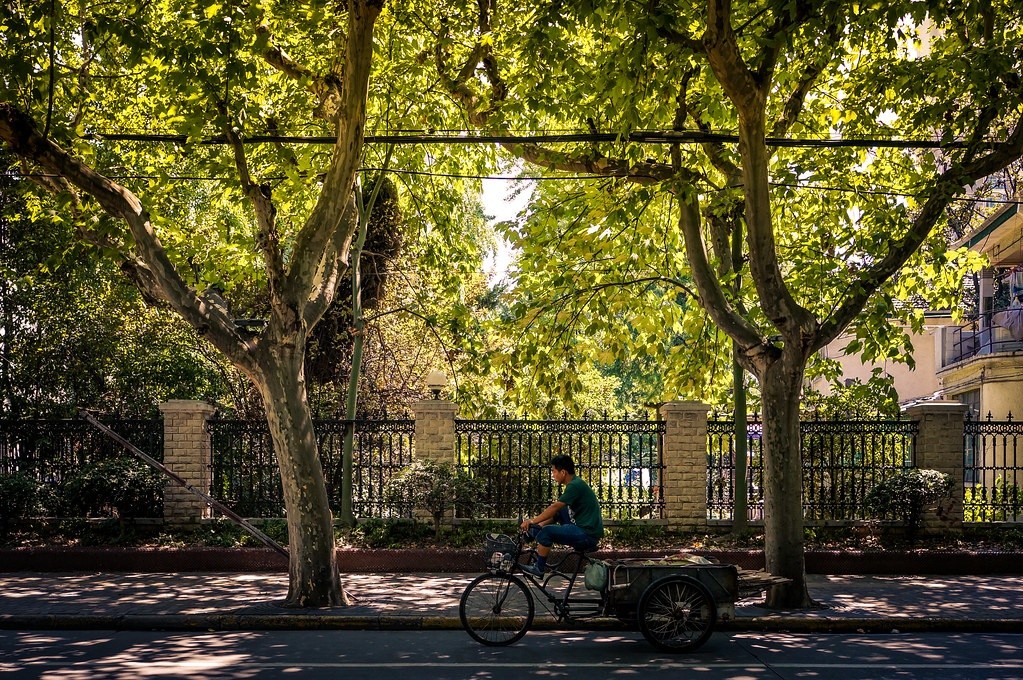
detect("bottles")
[499,553,512,572]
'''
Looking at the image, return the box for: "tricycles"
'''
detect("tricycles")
[459,522,776,656]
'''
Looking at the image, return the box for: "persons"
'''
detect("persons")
[518,454,604,580]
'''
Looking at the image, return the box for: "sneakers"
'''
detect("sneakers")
[517,516,534,545]
[517,562,544,579]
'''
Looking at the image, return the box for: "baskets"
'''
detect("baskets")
[485,534,516,571]
